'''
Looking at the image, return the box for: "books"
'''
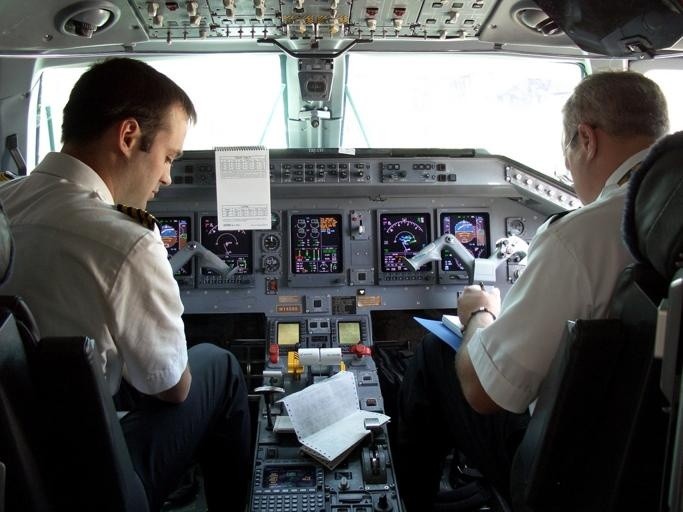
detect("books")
[441,314,466,341]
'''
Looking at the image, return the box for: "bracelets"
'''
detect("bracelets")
[459,308,496,329]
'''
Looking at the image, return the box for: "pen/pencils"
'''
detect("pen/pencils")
[479,281,486,291]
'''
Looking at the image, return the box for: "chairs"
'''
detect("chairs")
[1,206,209,511]
[426,131,681,511]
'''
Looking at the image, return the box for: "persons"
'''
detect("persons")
[396,72,671,494]
[1,58,252,504]
